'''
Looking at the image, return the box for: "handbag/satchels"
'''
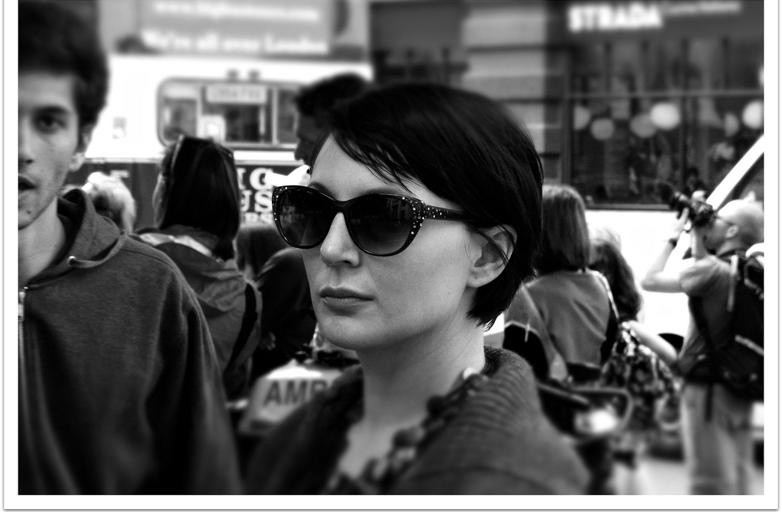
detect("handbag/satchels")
[595,322,679,429]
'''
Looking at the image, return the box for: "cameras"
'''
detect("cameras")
[669,191,714,228]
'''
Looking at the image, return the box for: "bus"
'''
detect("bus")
[332,0,764,352]
[63,53,376,233]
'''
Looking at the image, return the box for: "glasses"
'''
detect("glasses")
[270,183,485,257]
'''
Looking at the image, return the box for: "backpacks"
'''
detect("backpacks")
[688,250,763,401]
